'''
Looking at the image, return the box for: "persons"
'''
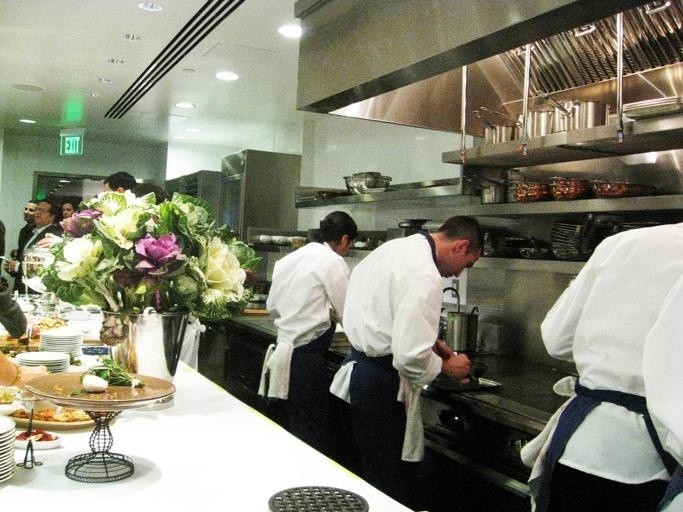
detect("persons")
[0,172,171,294]
[519,223,683,512]
[329,215,482,501]
[0,294,40,339]
[257,212,358,452]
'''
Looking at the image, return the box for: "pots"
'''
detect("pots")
[461,174,505,204]
[471,91,610,145]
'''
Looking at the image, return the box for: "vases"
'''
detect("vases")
[94,306,189,382]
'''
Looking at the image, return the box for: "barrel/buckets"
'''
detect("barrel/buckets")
[446,310,478,352]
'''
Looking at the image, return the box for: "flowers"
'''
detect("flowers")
[26,194,264,371]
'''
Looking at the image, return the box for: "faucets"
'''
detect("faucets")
[442,285,462,313]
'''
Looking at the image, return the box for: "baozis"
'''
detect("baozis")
[82,375,108,393]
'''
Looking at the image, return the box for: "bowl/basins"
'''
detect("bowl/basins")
[258,234,306,246]
[344,174,391,194]
[464,360,489,377]
[519,221,661,261]
[510,178,655,202]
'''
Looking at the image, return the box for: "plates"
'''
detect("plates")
[16,331,83,373]
[3,401,112,429]
[0,412,17,483]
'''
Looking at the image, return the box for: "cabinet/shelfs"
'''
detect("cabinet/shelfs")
[442,108,682,275]
